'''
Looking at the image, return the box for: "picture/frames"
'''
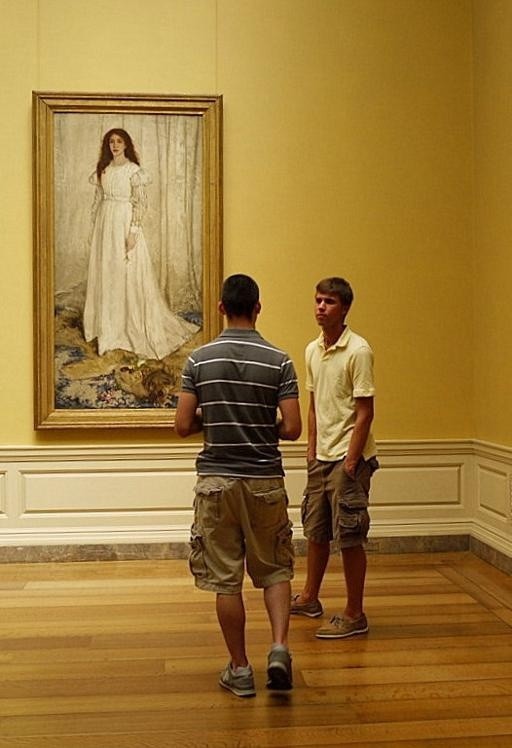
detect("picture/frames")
[32,91,223,429]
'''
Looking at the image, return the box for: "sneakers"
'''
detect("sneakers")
[289,594,323,617]
[218,662,255,696]
[265,646,293,690]
[315,613,369,638]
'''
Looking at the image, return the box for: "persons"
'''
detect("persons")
[286,277,377,642]
[84,127,200,360]
[175,272,304,698]
